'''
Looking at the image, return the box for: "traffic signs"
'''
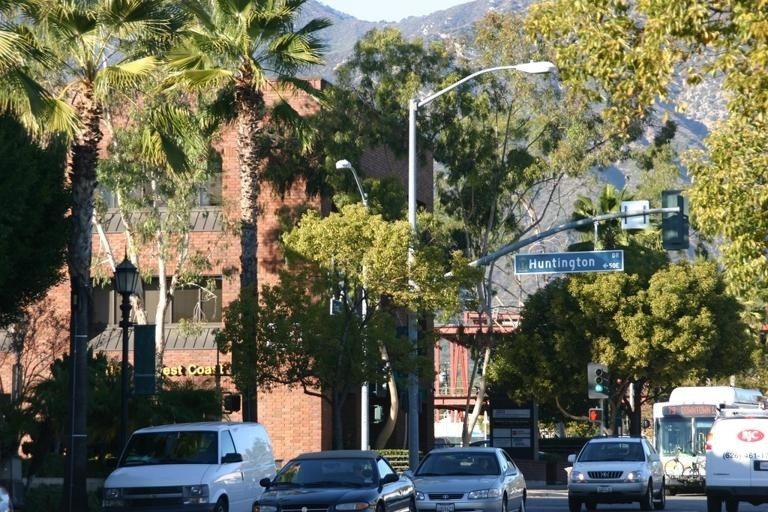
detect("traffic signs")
[515,250,624,275]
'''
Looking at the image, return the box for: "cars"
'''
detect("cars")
[253,450,414,512]
[411,446,527,512]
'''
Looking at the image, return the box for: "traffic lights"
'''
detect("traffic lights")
[595,369,608,393]
[589,407,602,422]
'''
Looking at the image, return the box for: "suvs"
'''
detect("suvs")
[568,437,666,512]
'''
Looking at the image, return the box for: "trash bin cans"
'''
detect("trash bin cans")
[547,453,560,484]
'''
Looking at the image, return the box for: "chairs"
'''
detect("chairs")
[431,458,496,473]
[297,468,371,481]
[589,447,642,458]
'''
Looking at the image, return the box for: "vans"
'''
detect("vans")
[103,422,277,512]
[704,398,767,511]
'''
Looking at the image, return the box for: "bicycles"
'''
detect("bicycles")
[663,448,706,477]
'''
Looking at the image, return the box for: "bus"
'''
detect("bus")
[653,384,763,495]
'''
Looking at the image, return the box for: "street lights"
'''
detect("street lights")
[408,61,558,449]
[335,157,371,450]
[109,246,139,455]
